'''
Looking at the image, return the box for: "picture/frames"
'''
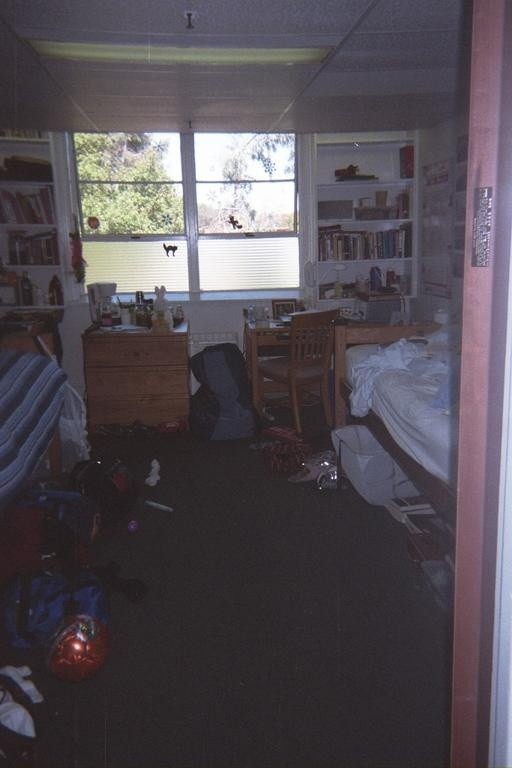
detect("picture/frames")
[272,299,296,320]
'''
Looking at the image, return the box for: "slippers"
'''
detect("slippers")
[288,461,344,503]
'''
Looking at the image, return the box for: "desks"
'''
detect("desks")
[243,317,291,422]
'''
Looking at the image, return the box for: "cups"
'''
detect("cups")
[375,190,387,207]
[253,305,265,320]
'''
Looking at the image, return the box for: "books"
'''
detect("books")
[399,145,414,179]
[317,191,412,262]
[1,183,59,265]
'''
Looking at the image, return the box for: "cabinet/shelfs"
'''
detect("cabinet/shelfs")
[313,135,420,326]
[0,127,66,312]
[78,318,193,434]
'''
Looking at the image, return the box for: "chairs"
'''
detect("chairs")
[259,307,341,434]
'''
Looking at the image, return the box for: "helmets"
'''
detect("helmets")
[46,615,111,681]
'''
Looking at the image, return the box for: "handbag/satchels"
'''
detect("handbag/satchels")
[0,574,104,650]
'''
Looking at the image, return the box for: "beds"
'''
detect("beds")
[0,331,64,512]
[332,320,461,497]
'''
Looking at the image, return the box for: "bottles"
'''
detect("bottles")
[369,266,382,291]
[21,271,33,306]
[36,288,46,305]
[129,291,153,327]
[248,304,255,322]
[97,296,121,326]
[386,268,395,287]
[7,235,28,266]
[49,274,63,305]
[168,304,185,329]
